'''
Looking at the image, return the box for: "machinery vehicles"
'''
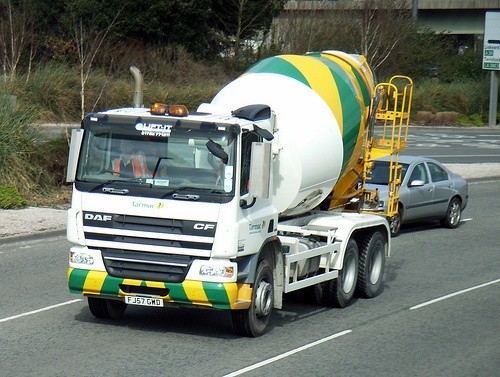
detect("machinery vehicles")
[64,48,414,339]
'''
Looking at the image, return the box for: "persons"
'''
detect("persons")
[218,165,250,190]
[111,136,158,178]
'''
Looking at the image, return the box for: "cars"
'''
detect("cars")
[357,153,469,238]
